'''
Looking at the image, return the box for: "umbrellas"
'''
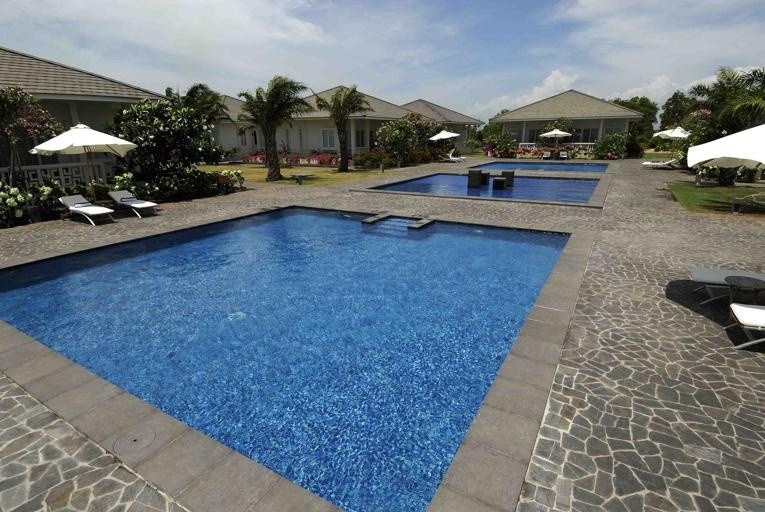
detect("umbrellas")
[686,124,765,170]
[653,126,690,147]
[539,129,572,147]
[29,122,138,201]
[427,130,461,154]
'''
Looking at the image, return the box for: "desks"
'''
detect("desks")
[95,200,113,207]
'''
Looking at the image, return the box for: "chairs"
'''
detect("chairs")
[438,153,467,162]
[723,303,765,349]
[640,158,676,168]
[108,190,158,219]
[560,152,568,160]
[543,152,551,160]
[686,268,765,306]
[58,195,115,227]
